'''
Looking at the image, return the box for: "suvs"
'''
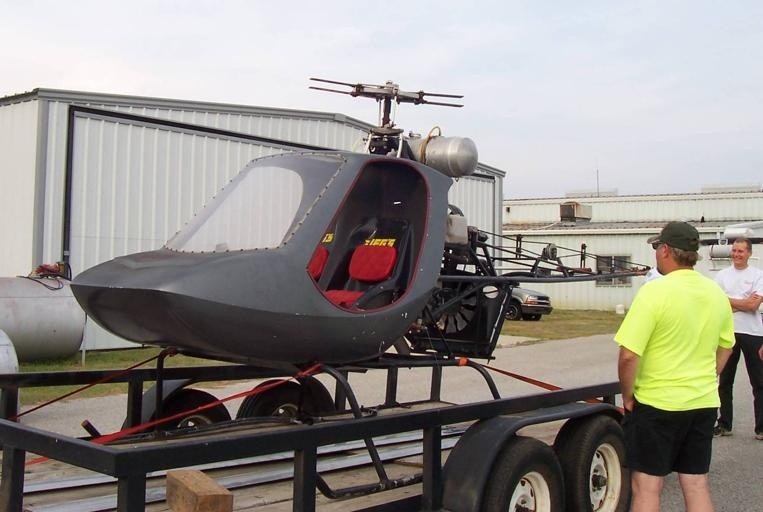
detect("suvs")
[444,214,469,244]
[616,304,625,315]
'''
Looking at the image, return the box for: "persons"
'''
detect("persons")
[758,345,763,360]
[613,221,737,512]
[711,239,763,439]
[641,268,661,282]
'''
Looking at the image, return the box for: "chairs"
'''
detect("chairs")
[712,425,733,438]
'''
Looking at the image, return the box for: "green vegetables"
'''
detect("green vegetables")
[70,75,651,501]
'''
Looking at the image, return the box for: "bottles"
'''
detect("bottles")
[646,220,699,251]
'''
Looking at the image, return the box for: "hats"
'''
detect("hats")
[504,285,552,321]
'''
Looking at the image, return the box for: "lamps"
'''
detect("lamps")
[306,218,415,312]
